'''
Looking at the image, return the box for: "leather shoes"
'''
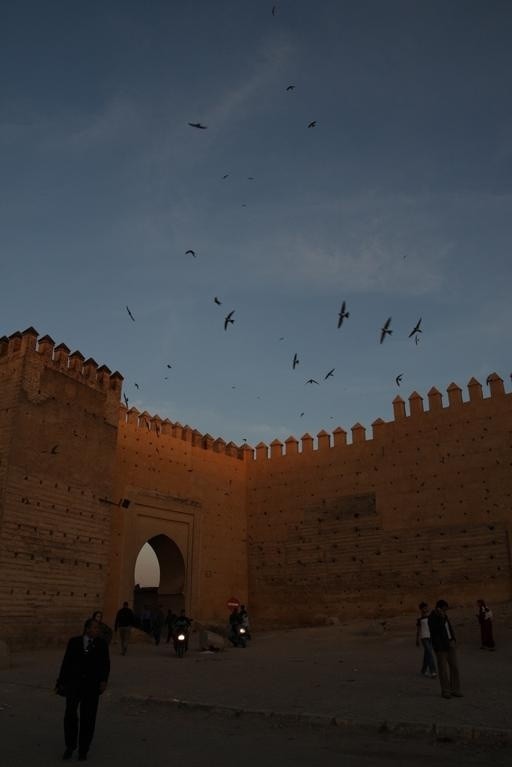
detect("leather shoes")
[64,745,76,760]
[79,753,87,762]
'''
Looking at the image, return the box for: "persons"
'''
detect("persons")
[429,600,464,698]
[91,611,112,646]
[416,602,439,679]
[54,619,110,762]
[477,600,496,651]
[113,601,251,654]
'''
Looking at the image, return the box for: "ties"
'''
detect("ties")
[87,639,92,651]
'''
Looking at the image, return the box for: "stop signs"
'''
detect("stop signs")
[225,598,239,612]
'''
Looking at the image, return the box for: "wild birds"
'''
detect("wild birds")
[222,309,234,331]
[188,120,208,132]
[123,392,130,409]
[213,295,221,307]
[414,335,420,346]
[307,119,315,130]
[287,84,294,90]
[303,378,320,387]
[408,317,423,339]
[324,367,335,380]
[336,300,350,330]
[290,352,298,370]
[183,247,198,259]
[126,305,136,324]
[271,5,278,19]
[379,316,392,345]
[395,371,404,388]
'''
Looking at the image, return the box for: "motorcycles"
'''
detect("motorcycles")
[173,627,188,657]
[228,624,251,649]
